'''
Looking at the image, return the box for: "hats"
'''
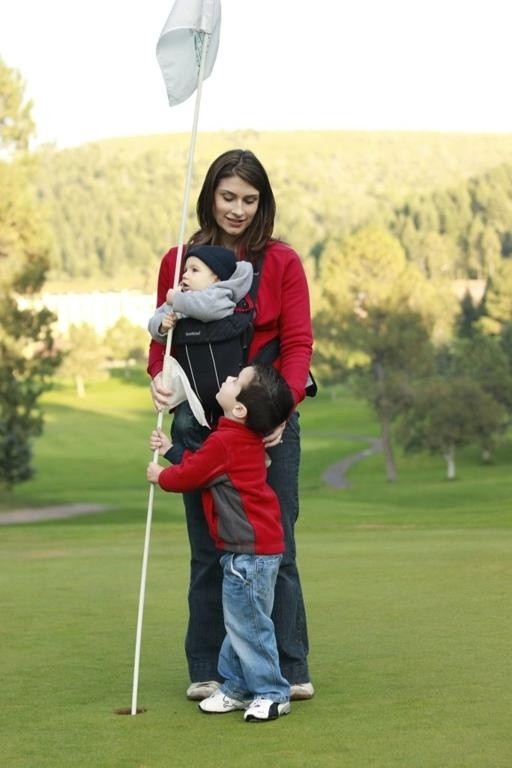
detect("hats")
[184,244,238,282]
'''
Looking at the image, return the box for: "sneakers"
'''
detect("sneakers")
[197,686,253,714]
[187,680,220,699]
[242,694,292,722]
[289,682,314,701]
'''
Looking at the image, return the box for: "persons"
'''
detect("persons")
[146,243,274,469]
[155,0,222,108]
[145,147,317,701]
[144,360,292,723]
